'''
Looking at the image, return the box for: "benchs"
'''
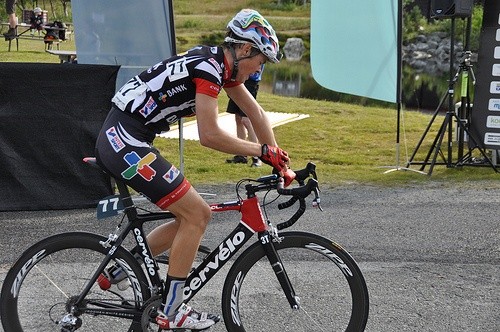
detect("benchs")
[0,34,63,53]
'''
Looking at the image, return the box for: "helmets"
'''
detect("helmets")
[227,9,280,63]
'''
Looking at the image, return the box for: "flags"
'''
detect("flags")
[71,0,172,66]
[309,0,398,103]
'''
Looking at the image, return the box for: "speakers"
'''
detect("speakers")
[430,0,472,21]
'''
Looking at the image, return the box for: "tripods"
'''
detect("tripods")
[419,17,500,176]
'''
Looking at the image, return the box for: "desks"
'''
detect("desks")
[46,50,77,64]
[0,23,68,52]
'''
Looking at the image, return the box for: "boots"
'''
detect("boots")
[38,30,41,36]
[3,27,15,38]
[30,29,34,35]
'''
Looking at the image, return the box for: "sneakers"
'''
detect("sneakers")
[97,264,133,290]
[226,154,247,163]
[251,156,262,167]
[155,302,221,330]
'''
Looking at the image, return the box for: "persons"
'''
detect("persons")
[96,8,296,332]
[30,7,43,36]
[44,29,59,51]
[225,63,265,167]
[3,0,18,41]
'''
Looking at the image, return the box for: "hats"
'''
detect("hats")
[34,7,42,13]
